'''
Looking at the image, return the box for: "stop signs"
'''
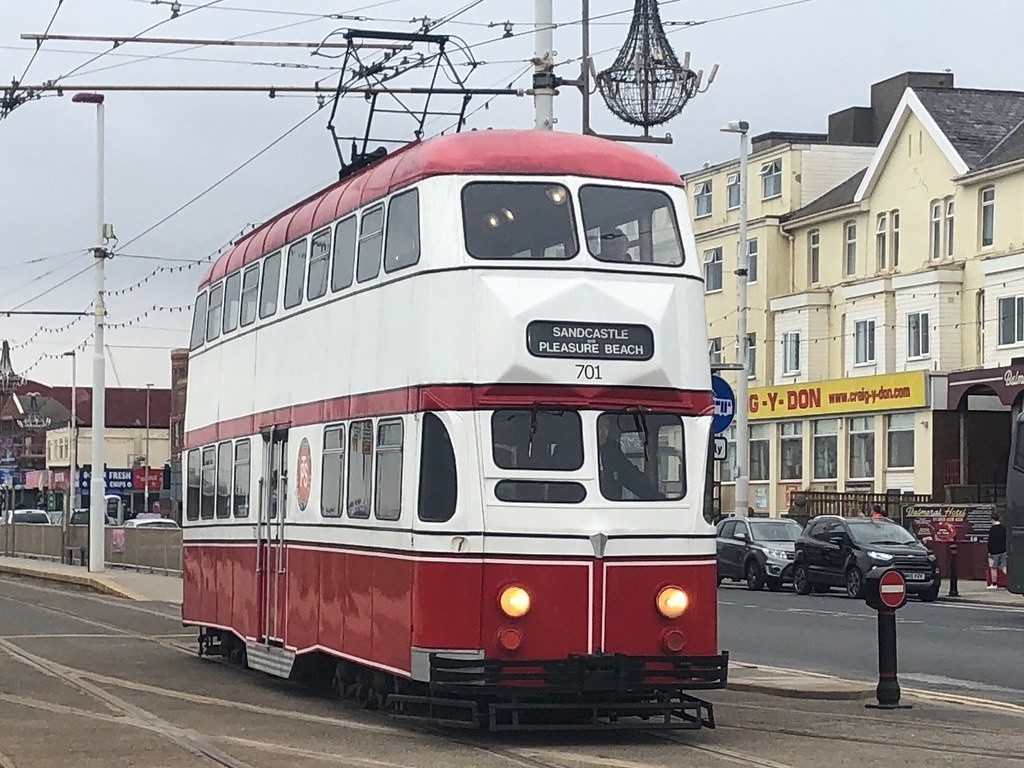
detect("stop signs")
[879,571,907,607]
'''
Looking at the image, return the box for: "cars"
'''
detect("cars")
[123,518,178,529]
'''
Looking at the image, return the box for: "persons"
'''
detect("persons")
[748,506,753,518]
[871,504,883,518]
[786,493,810,529]
[600,230,633,260]
[526,413,671,506]
[986,513,1008,590]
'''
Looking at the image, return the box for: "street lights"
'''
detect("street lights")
[71,94,107,575]
[721,121,751,521]
[143,384,155,512]
[63,351,77,524]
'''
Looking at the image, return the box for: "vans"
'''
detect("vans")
[58,507,111,526]
[5,509,51,525]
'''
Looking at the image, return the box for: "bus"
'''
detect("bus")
[181,30,729,735]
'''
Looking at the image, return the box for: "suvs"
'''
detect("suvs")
[714,515,805,591]
[794,515,939,602]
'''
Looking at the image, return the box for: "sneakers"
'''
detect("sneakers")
[987,584,997,590]
